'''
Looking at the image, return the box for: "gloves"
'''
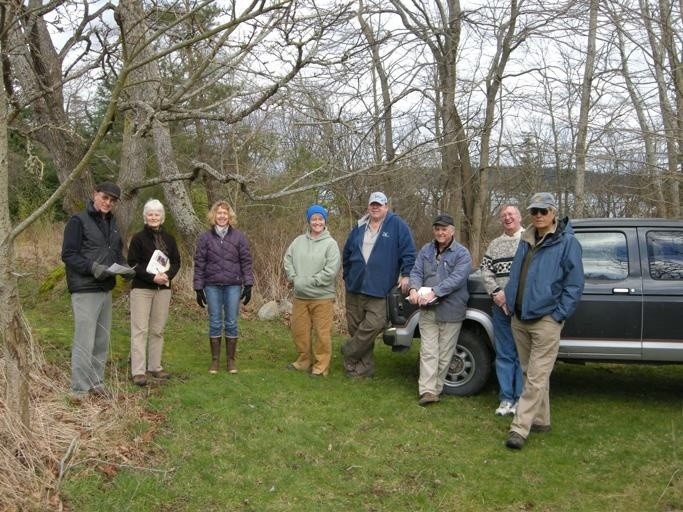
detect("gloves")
[197,290,206,308]
[240,285,251,305]
[119,263,136,279]
[91,262,113,280]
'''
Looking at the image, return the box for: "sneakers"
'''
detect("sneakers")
[287,345,374,379]
[418,393,440,405]
[133,375,146,385]
[495,400,551,448]
[148,369,169,379]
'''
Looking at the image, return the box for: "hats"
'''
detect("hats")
[96,182,121,201]
[368,192,387,205]
[432,215,454,227]
[526,193,556,210]
[307,205,326,224]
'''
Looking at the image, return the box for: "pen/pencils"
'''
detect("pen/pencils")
[156,268,161,275]
[131,264,137,270]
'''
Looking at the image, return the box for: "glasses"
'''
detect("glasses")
[530,208,547,215]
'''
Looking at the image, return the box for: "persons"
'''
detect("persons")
[59,180,139,402]
[406,214,473,406]
[341,192,417,379]
[191,200,254,374]
[503,192,586,450]
[282,204,341,377]
[127,199,180,386]
[479,204,525,416]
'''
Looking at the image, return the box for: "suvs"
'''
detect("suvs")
[383,216,683,397]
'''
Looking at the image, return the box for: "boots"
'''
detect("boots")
[208,336,221,375]
[225,335,238,373]
